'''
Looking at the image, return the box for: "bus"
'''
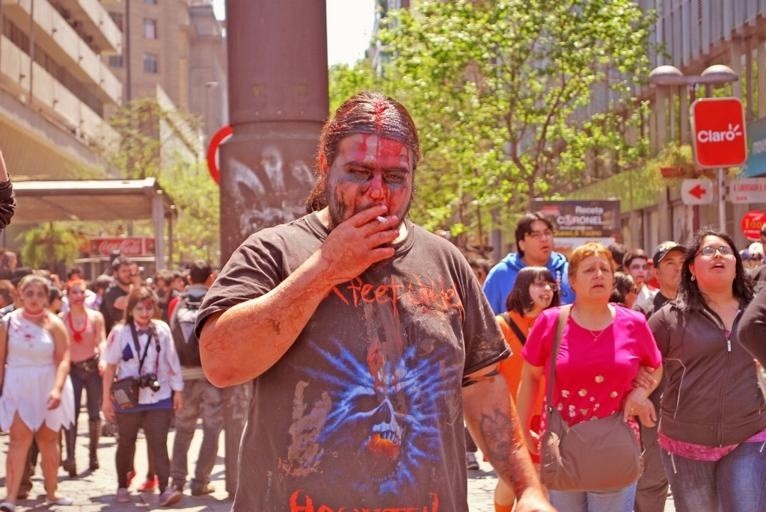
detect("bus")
[77,257,170,285]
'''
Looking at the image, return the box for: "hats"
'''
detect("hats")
[653,240,688,268]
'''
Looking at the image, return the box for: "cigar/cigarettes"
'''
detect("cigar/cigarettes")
[376,215,389,224]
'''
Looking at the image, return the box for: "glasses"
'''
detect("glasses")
[532,282,558,291]
[699,245,732,257]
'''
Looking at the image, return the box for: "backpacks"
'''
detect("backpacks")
[170,291,205,368]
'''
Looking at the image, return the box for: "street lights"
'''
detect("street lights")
[199,79,219,171]
[652,61,739,239]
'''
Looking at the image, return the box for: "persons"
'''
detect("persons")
[193,88,556,512]
[431,211,766,512]
[0,149,253,512]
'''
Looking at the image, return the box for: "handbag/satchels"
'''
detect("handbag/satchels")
[538,403,645,492]
[112,376,140,409]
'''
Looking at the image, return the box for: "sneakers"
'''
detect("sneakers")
[117,471,216,506]
[0,501,15,512]
[466,451,479,470]
[45,497,73,506]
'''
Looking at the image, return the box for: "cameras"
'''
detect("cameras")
[139,373,160,392]
